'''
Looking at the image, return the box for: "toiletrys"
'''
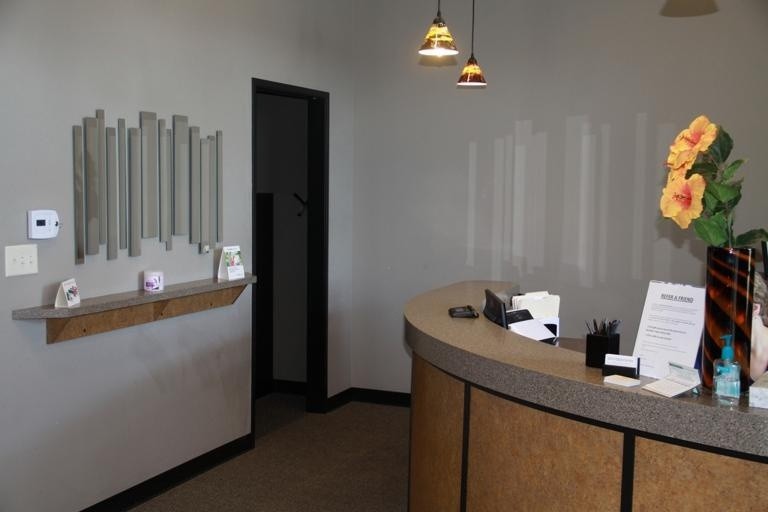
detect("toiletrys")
[712,335,741,398]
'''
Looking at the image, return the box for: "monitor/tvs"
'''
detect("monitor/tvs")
[484,290,508,329]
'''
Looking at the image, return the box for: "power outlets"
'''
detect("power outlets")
[5,244,38,277]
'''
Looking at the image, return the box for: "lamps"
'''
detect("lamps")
[456,0,489,86]
[417,0,459,56]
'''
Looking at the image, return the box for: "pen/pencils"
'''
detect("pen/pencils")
[467,304,479,318]
[585,319,613,334]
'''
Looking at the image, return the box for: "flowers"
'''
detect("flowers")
[661,116,768,249]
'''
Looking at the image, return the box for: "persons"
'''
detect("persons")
[750,271,768,389]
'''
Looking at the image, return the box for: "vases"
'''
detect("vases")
[702,245,754,392]
